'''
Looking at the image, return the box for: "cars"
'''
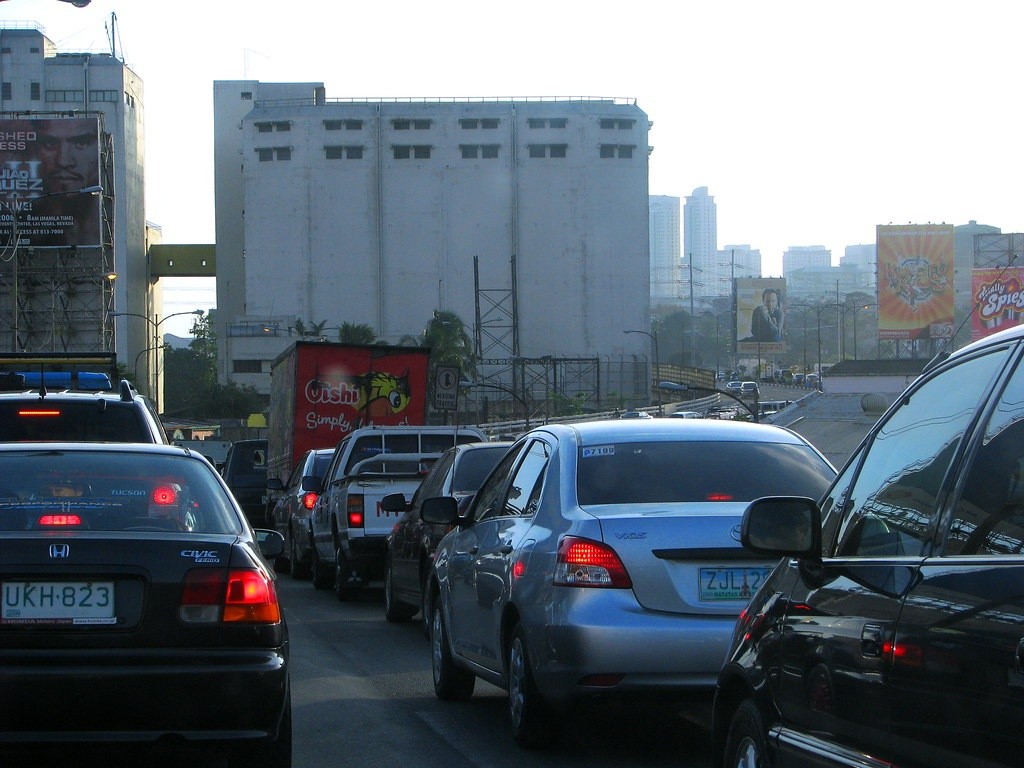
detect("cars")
[420,416,893,747]
[1,442,296,767]
[377,439,514,641]
[619,368,819,422]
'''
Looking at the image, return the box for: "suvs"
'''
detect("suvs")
[0,374,218,531]
[263,448,339,580]
[711,324,1023,767]
[219,441,267,528]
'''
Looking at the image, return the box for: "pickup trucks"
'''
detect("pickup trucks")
[300,424,491,599]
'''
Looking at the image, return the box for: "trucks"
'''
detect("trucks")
[264,339,430,525]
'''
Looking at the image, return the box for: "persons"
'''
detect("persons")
[21,118,99,245]
[738,288,784,341]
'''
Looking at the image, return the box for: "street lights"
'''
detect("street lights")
[1,270,118,360]
[456,380,530,432]
[134,341,170,389]
[663,314,702,367]
[623,328,663,418]
[739,297,878,388]
[109,309,204,415]
[659,380,760,423]
[441,317,505,428]
[700,308,736,381]
[0,184,104,353]
